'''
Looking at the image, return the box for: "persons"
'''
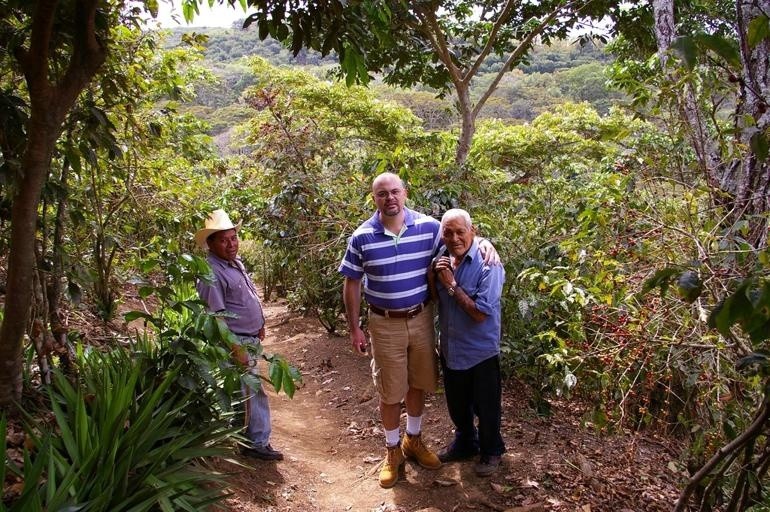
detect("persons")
[195,207,284,461]
[427,206,507,477]
[333,170,501,487]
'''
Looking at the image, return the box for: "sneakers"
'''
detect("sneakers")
[474,455,501,476]
[437,445,478,463]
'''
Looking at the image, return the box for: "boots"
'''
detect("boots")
[378,441,405,488]
[402,430,441,470]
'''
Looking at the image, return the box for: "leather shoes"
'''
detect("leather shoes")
[240,444,283,460]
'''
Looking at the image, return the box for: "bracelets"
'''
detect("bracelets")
[477,235,488,246]
[447,281,456,299]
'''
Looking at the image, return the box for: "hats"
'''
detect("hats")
[194,208,243,250]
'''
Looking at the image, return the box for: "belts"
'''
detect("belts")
[370,297,430,319]
